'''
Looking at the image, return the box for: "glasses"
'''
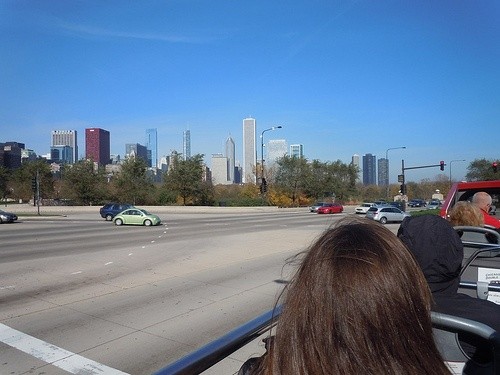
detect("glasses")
[486,203,493,212]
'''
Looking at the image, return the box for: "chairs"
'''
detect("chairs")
[452,225,500,270]
[129,210,133,215]
[456,242,500,306]
[430,310,497,375]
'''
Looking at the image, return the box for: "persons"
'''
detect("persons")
[447,201,480,227]
[473,192,500,229]
[238,215,453,375]
[397,214,500,358]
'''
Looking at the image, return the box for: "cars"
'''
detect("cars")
[0,209,18,224]
[317,202,343,214]
[309,201,330,212]
[429,198,442,205]
[112,207,161,227]
[355,199,427,224]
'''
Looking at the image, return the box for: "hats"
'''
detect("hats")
[397,212,465,295]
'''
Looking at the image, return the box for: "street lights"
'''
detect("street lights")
[385,146,406,198]
[261,125,283,193]
[450,159,466,188]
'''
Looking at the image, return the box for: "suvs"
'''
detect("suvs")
[100,202,133,221]
[437,180,500,233]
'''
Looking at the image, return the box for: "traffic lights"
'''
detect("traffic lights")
[400,185,404,193]
[261,178,267,193]
[492,161,498,173]
[440,160,445,170]
[32,177,37,190]
